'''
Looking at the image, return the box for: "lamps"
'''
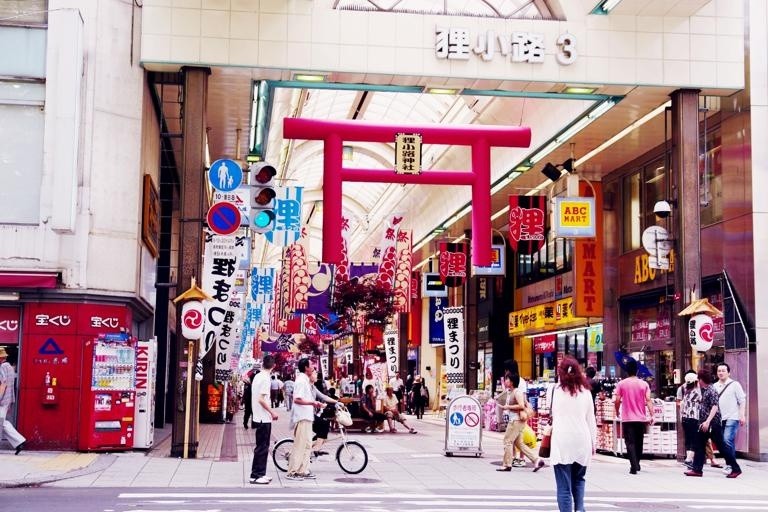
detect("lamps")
[538,158,574,181]
[651,195,678,220]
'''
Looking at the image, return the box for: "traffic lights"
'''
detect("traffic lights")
[250,161,277,233]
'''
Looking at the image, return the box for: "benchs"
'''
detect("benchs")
[322,396,398,433]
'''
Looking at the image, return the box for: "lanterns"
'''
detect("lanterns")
[181,300,204,339]
[689,313,713,353]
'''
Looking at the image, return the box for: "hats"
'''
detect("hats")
[0,348,8,358]
[413,377,422,384]
[685,372,697,384]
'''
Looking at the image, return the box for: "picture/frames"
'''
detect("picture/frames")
[140,171,163,260]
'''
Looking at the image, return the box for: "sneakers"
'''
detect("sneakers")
[390,428,396,433]
[408,428,416,434]
[495,458,544,473]
[364,426,371,433]
[313,450,328,457]
[686,470,702,476]
[373,427,384,433]
[249,473,316,485]
[727,470,741,478]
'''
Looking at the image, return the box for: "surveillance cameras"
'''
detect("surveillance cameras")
[652,200,672,219]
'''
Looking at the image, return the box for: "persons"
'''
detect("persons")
[677,364,745,478]
[494,360,528,467]
[0,348,26,455]
[249,356,278,484]
[241,371,294,428]
[289,367,339,456]
[321,372,428,433]
[496,372,544,472]
[286,358,322,481]
[546,357,597,512]
[583,367,605,415]
[616,362,654,474]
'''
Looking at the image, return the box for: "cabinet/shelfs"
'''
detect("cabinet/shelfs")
[591,390,683,461]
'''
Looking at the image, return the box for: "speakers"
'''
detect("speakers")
[541,162,562,181]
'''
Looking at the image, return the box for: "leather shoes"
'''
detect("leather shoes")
[15,441,25,455]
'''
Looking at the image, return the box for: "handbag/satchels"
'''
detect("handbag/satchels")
[334,406,352,427]
[515,390,536,421]
[538,385,555,458]
[419,385,428,398]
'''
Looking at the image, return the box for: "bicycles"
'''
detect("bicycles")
[238,392,245,410]
[272,403,368,474]
